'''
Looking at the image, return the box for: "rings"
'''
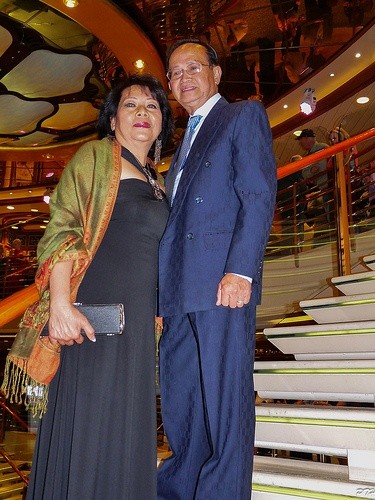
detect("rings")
[236,300,245,305]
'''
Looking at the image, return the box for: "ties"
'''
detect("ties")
[167,115,205,208]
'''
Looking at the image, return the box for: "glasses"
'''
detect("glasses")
[166,61,213,81]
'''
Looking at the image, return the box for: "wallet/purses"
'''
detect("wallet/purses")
[42,304,125,336]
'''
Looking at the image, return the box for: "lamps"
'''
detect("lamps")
[300,88,316,115]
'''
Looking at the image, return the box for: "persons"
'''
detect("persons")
[25,72,174,500]
[156,37,278,500]
[181,0,370,104]
[8,240,30,270]
[326,124,362,221]
[298,127,334,225]
[277,149,313,248]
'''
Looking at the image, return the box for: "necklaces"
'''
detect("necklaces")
[117,146,167,201]
